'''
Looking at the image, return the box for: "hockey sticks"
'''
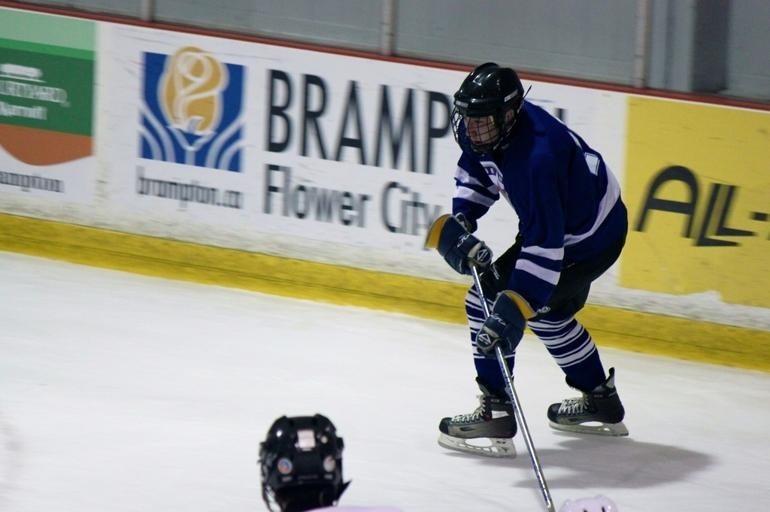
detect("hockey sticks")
[466,257,616,512]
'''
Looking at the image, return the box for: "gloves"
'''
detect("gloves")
[425,211,491,274]
[475,290,536,359]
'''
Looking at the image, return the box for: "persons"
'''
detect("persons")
[423,61,629,439]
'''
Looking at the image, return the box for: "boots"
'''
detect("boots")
[548,367,624,426]
[439,377,517,438]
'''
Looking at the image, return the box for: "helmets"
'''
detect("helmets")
[261,415,350,512]
[447,63,524,156]
